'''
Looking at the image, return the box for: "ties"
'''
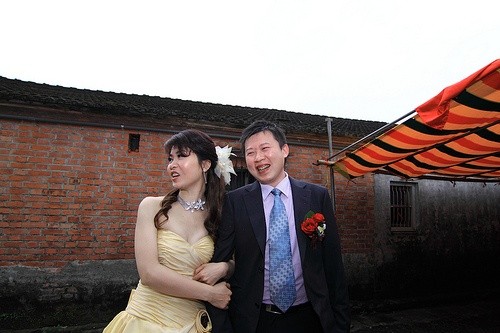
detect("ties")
[268,189,296,313]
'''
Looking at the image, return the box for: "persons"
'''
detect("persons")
[203,122,352,333]
[103,130,234,333]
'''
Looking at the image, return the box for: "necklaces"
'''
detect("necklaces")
[177,194,206,213]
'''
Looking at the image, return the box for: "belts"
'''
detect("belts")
[261,301,311,314]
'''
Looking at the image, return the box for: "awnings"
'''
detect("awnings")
[316,57,500,214]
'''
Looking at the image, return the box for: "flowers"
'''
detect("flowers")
[301,211,327,251]
[213,145,236,185]
